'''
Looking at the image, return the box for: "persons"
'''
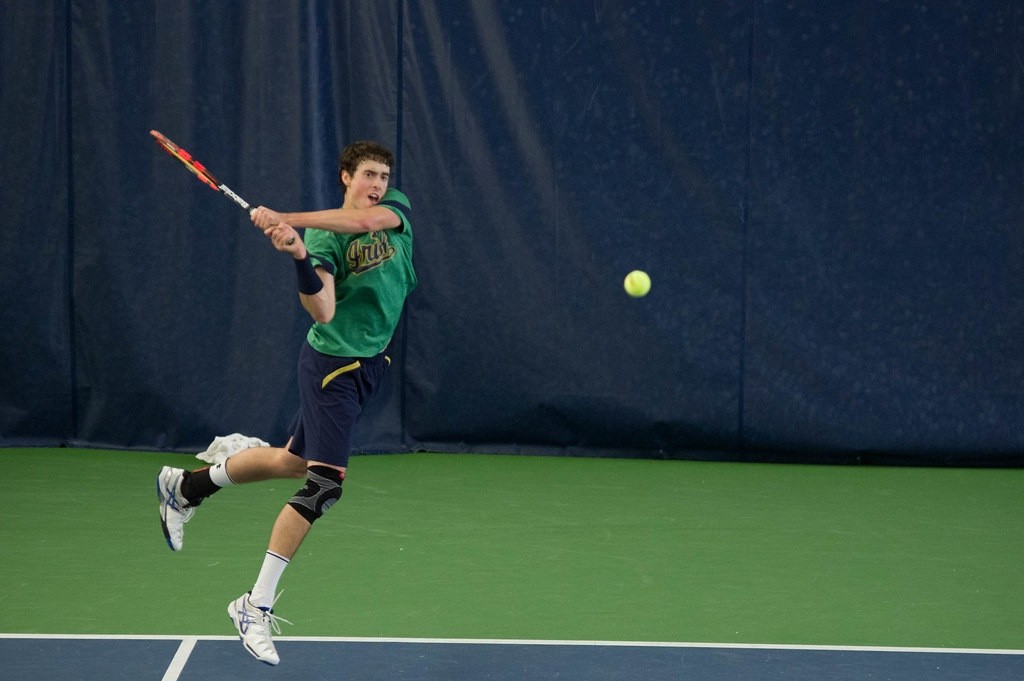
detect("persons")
[157,142,418,667]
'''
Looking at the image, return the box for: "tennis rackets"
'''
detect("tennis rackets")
[149,129,295,246]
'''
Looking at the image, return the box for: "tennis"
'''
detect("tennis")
[622,270,653,299]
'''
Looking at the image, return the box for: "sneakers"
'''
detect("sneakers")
[156,466,195,552]
[227,590,280,667]
